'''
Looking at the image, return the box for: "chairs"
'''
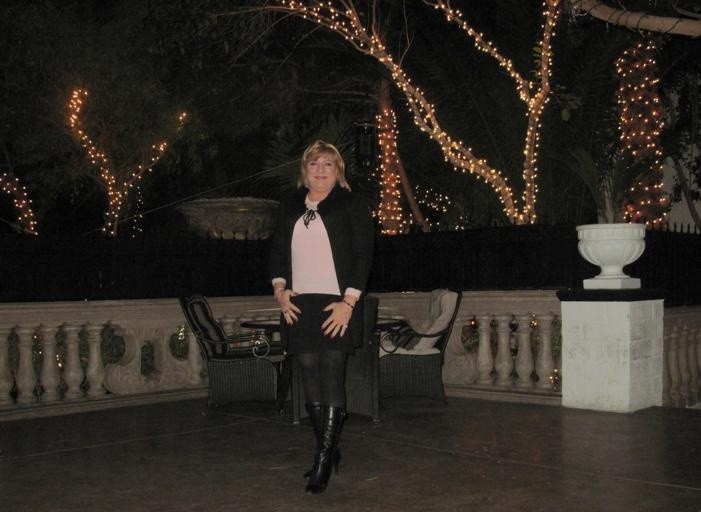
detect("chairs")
[178,292,290,418]
[378,289,463,406]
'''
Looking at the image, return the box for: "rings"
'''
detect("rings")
[282,311,288,315]
[343,324,348,328]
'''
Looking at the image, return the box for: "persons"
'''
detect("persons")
[263,139,377,495]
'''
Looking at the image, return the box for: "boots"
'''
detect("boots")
[303,401,346,494]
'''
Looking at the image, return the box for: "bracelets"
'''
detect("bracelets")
[341,298,356,310]
[274,286,284,300]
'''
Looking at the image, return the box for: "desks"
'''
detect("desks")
[239,317,404,418]
